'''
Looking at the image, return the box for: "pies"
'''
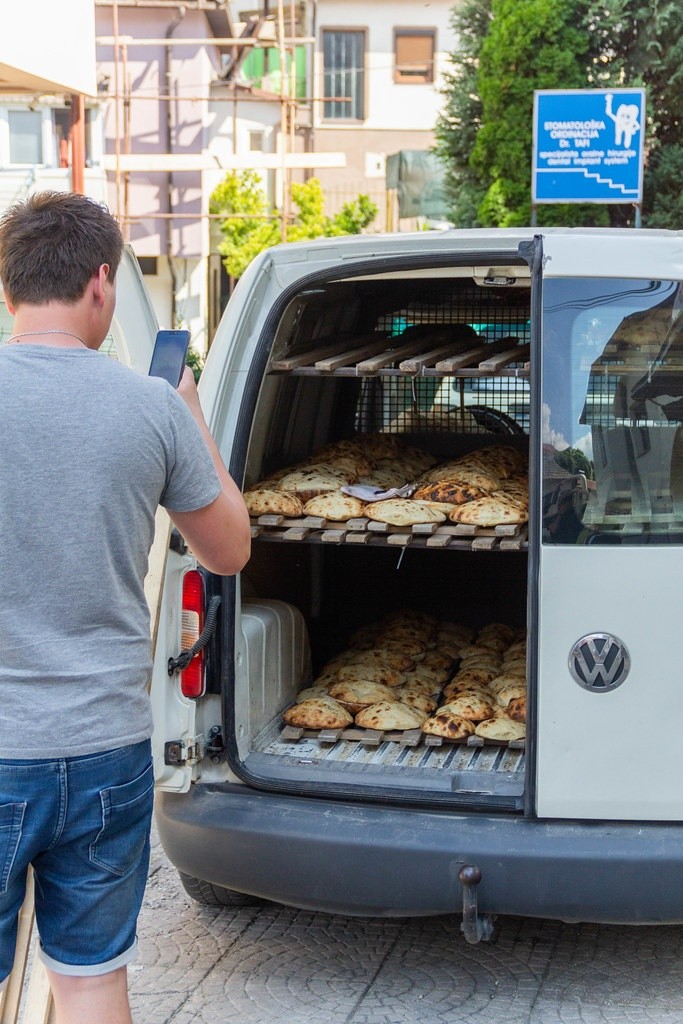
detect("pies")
[242,435,529,526]
[284,611,529,742]
[604,308,683,346]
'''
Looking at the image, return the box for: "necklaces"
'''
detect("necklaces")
[4,330,86,345]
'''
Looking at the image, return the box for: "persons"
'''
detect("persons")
[0,190,253,1024]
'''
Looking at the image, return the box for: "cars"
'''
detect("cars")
[430,322,681,435]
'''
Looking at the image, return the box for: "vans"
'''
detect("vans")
[0,225,683,945]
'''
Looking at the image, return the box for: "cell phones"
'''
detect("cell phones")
[148,330,191,389]
